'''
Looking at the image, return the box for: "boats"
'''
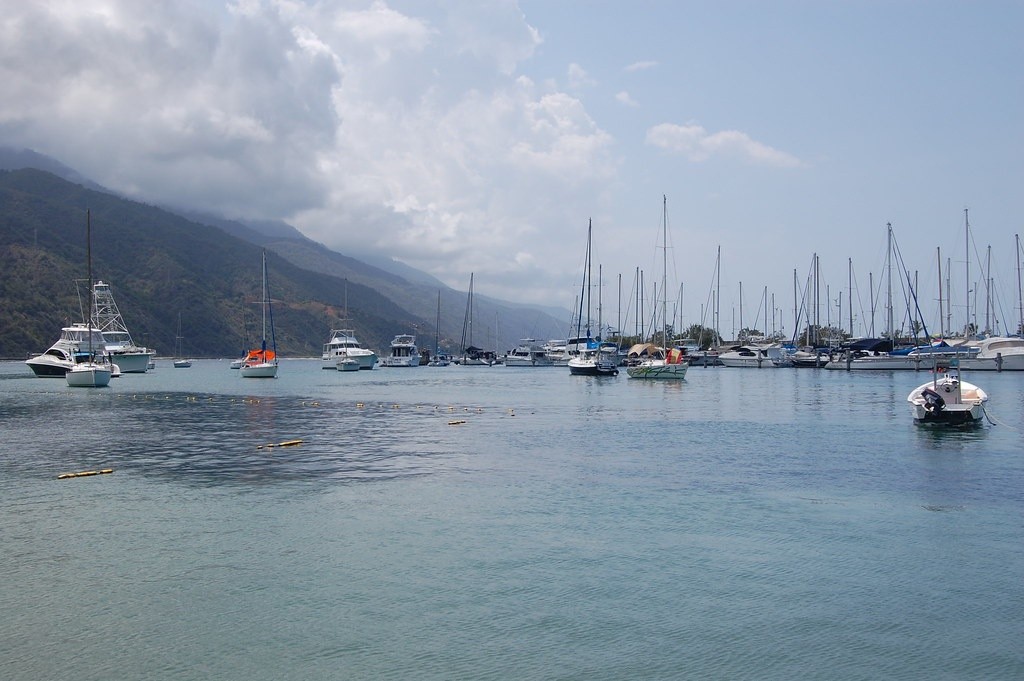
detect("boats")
[420,347,432,366]
[25,280,158,377]
[907,349,987,425]
[377,334,422,367]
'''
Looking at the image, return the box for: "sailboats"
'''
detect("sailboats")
[322,318,378,369]
[428,194,1024,381]
[173,311,193,369]
[65,209,113,389]
[228,294,250,369]
[240,246,279,379]
[335,278,361,371]
